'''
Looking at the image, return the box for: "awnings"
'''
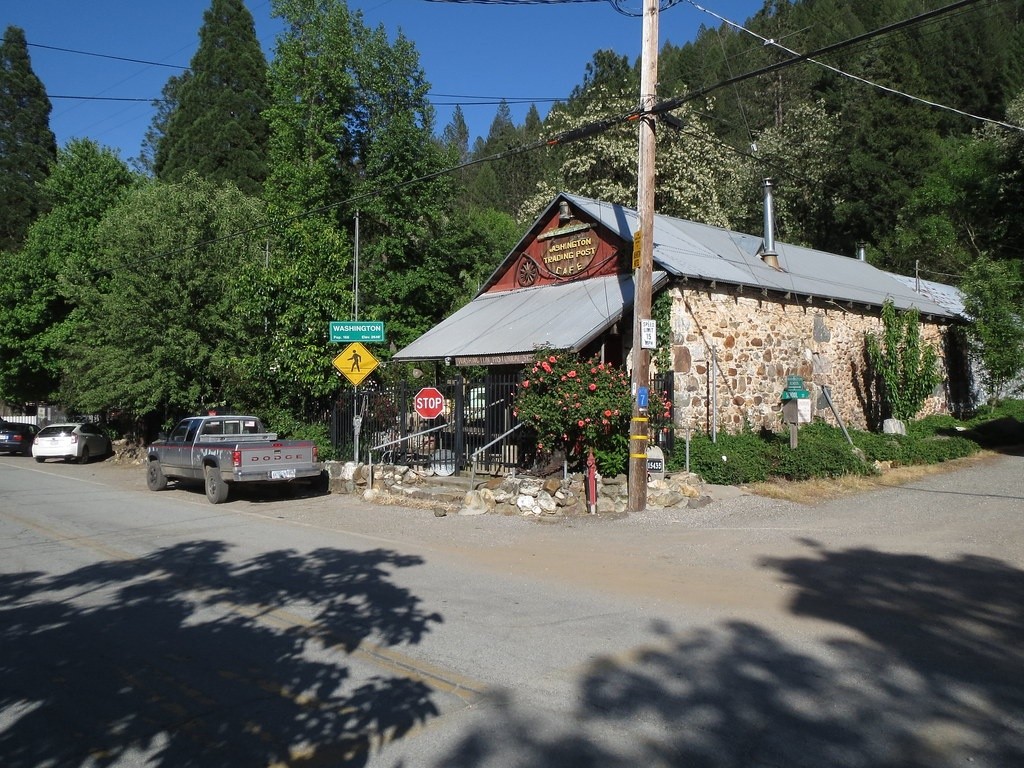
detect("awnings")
[392,271,669,366]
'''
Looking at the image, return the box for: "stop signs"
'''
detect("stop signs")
[413,388,445,420]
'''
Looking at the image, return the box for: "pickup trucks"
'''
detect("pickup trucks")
[146,415,321,505]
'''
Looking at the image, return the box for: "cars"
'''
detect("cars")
[0,422,41,455]
[31,422,112,465]
